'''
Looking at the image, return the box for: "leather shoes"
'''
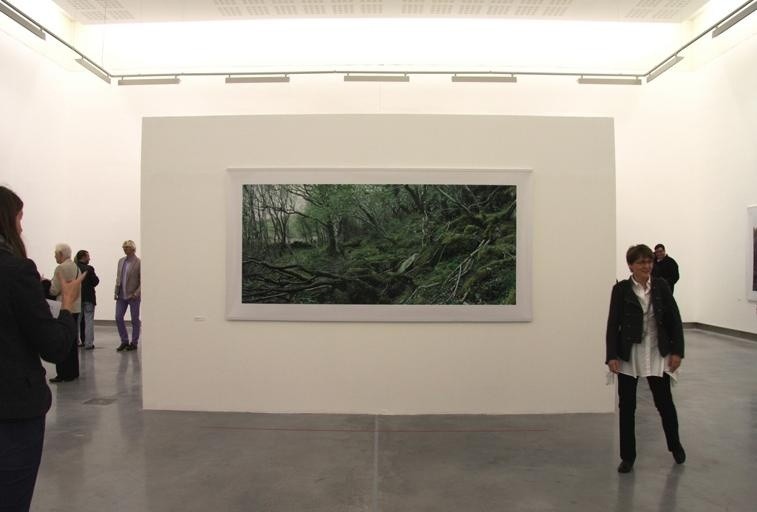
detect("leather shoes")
[49,372,79,383]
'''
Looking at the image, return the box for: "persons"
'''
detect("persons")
[651,244,680,293]
[76,250,99,350]
[1,184,87,511]
[113,240,140,352]
[41,242,83,381]
[606,243,687,475]
[74,254,85,347]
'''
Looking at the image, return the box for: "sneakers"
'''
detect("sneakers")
[617,457,636,472]
[125,343,137,350]
[78,341,95,349]
[116,343,129,351]
[673,444,686,464]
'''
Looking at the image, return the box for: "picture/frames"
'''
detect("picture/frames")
[224,167,533,326]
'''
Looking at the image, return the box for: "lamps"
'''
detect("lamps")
[75,57,111,83]
[226,73,289,82]
[0,0,46,42]
[344,73,409,82]
[119,76,180,85]
[577,52,683,88]
[710,0,757,40]
[452,74,515,82]
